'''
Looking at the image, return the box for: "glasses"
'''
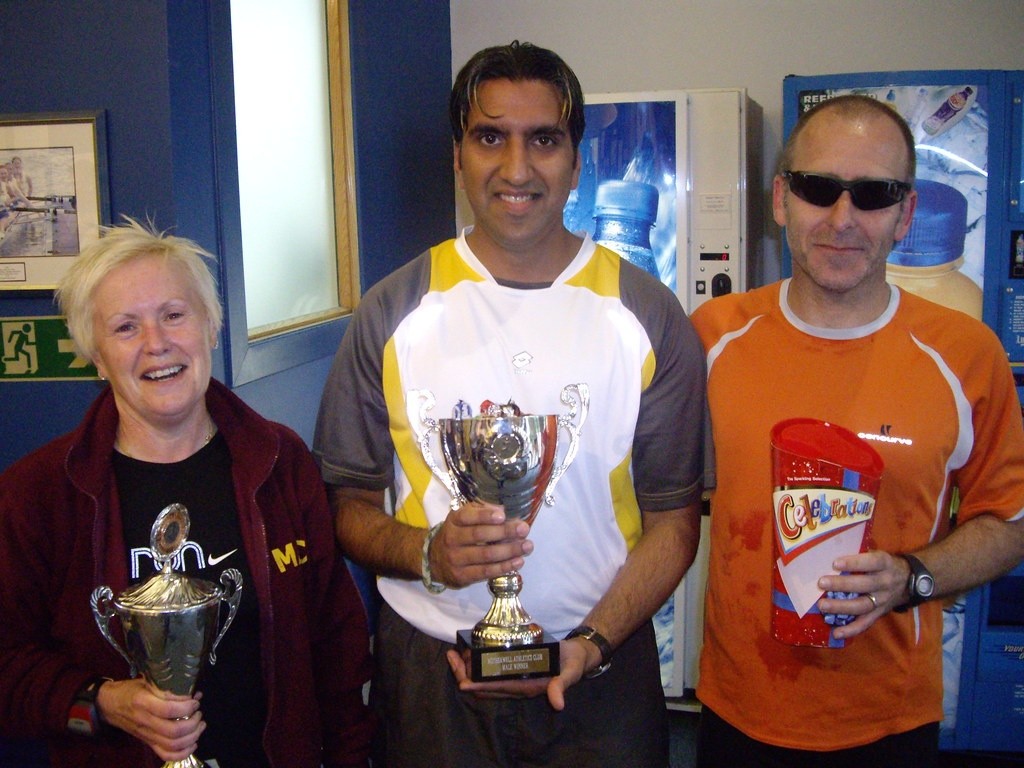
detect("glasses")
[783,171,909,210]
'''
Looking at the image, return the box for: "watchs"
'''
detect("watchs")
[565,625,613,680]
[66,679,113,737]
[894,554,935,613]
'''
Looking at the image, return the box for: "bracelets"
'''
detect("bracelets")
[421,522,446,594]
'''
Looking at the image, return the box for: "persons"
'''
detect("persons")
[0,211,376,768]
[689,96,1023,768]
[0,157,32,210]
[312,44,706,768]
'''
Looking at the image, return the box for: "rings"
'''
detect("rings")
[868,594,877,610]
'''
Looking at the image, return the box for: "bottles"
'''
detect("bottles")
[883,89,898,112]
[921,86,974,135]
[885,180,983,324]
[590,178,662,283]
[903,88,929,133]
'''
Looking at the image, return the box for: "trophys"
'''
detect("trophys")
[406,383,590,682]
[90,504,243,768]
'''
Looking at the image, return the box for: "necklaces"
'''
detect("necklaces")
[118,419,214,458]
